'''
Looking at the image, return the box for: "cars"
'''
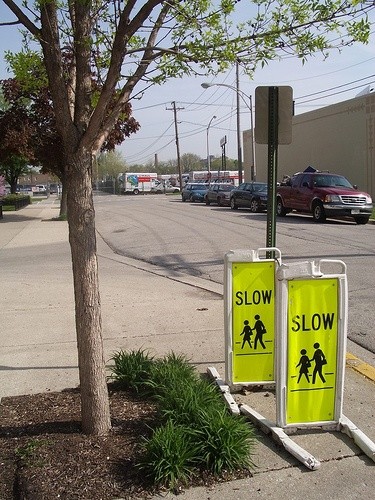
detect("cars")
[230,182,267,212]
[151,183,180,194]
[35,185,46,192]
[204,183,237,207]
[3,186,11,194]
[182,183,208,202]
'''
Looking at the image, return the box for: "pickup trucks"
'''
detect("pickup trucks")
[277,170,373,224]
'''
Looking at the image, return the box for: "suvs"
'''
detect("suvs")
[50,183,60,195]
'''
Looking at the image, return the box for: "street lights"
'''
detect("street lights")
[206,115,217,181]
[201,81,256,182]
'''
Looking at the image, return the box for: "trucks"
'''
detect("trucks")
[122,172,158,195]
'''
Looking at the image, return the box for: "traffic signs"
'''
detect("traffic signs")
[220,135,226,146]
